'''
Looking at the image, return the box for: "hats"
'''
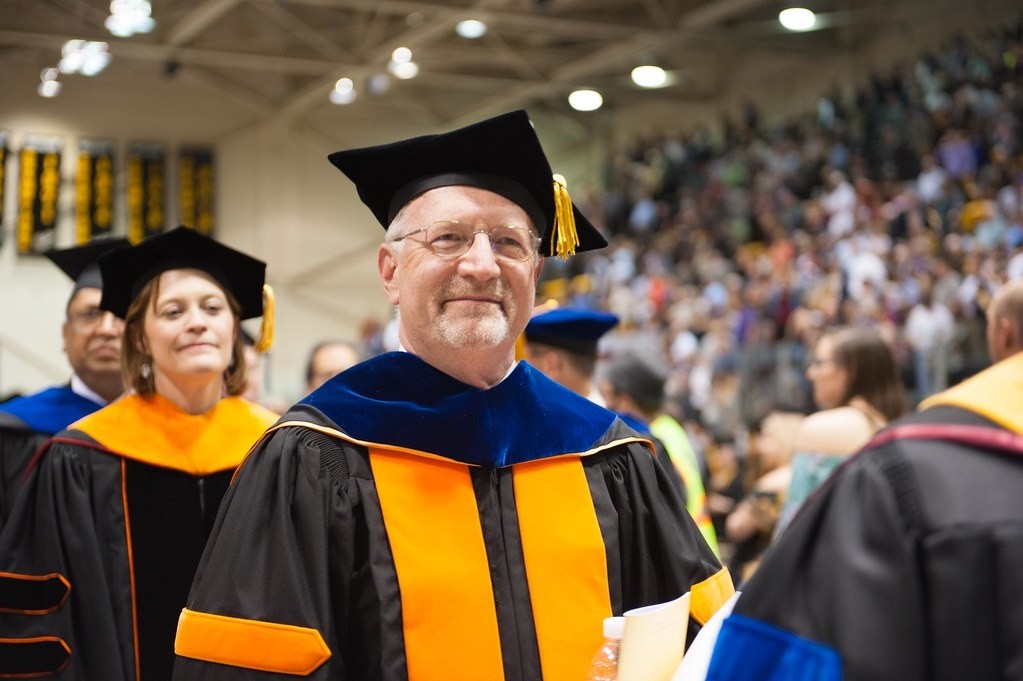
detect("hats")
[525,308,620,352]
[328,110,608,261]
[44,237,132,294]
[96,225,275,352]
[603,347,666,402]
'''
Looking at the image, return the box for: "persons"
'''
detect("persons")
[669,351,1023,681]
[172,107,736,681]
[0,224,282,681]
[305,27,1023,592]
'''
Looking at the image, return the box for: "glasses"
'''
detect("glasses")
[393,220,542,263]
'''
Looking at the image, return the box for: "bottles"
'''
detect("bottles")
[591,616,626,681]
